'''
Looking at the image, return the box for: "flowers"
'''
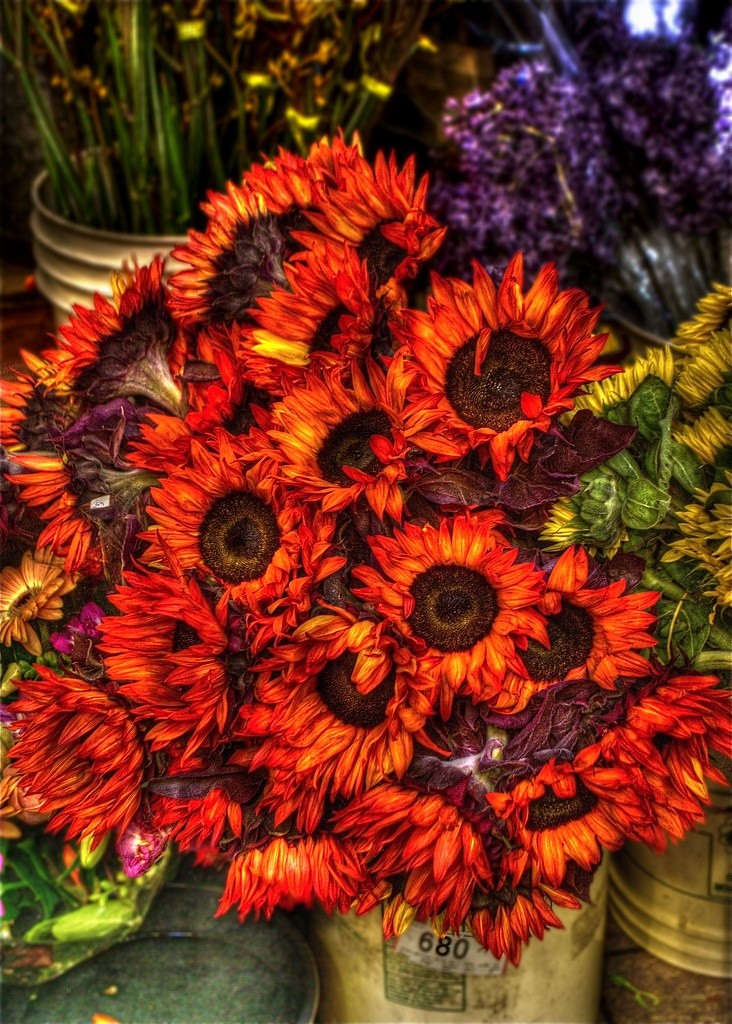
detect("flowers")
[0,0,732,973]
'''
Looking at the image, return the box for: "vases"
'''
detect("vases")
[32,140,196,347]
[607,752,732,979]
[316,847,607,1024]
